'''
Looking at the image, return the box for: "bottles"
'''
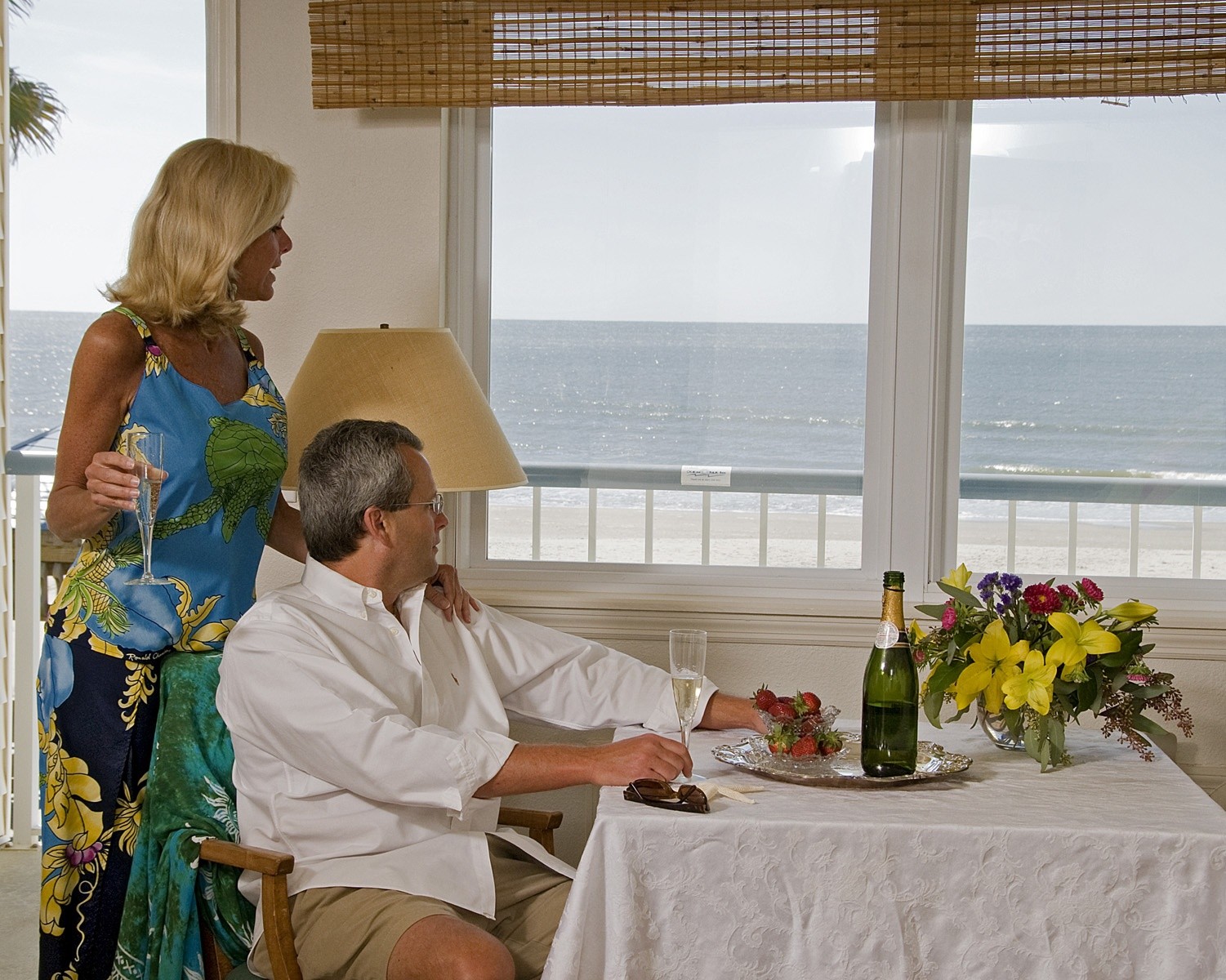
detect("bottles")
[860,571,919,777]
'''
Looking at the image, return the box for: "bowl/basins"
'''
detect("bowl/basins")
[750,742,852,771]
[751,705,840,734]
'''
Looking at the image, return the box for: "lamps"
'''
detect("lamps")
[275,322,528,493]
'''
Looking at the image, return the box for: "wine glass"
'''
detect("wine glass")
[123,433,171,586]
[669,629,707,785]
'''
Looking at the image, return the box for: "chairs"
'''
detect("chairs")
[111,651,579,979]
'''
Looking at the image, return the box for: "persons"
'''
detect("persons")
[214,419,771,980]
[37,137,481,980]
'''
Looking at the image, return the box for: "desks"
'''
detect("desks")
[538,717,1224,977]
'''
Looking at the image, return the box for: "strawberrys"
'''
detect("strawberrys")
[750,683,848,758]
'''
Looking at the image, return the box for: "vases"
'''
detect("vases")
[980,697,1058,751]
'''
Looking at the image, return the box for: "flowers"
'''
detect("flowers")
[911,564,1194,774]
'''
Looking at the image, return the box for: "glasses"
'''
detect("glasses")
[358,494,445,528]
[622,778,710,814]
